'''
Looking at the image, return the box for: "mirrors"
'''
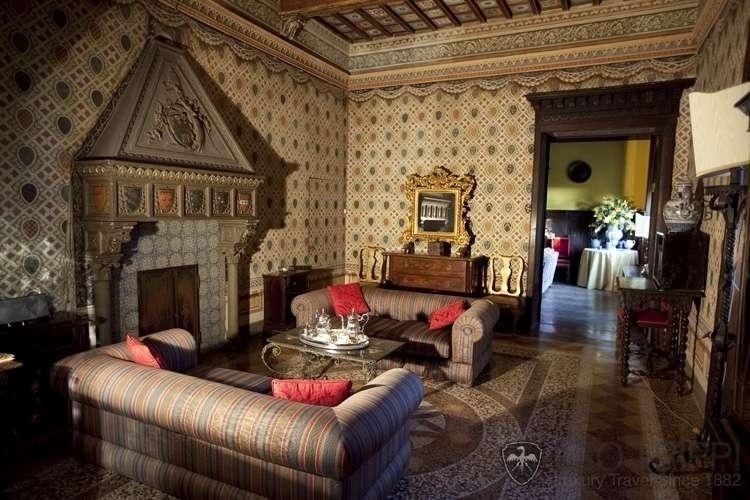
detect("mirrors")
[397,166,478,250]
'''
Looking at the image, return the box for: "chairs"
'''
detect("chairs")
[481,253,524,339]
[551,234,572,284]
[357,246,387,287]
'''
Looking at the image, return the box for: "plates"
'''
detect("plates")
[300,328,369,350]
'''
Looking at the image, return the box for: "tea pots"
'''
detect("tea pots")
[590,238,636,249]
[339,308,369,333]
[315,307,332,334]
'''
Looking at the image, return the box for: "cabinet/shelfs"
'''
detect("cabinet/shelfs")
[262,267,335,341]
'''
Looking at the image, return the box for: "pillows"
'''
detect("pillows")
[271,376,353,408]
[126,332,172,372]
[327,282,371,318]
[428,298,471,330]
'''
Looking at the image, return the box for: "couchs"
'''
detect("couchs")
[289,281,501,390]
[52,327,425,500]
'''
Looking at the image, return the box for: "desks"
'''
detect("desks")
[615,275,705,398]
[577,248,639,293]
[381,251,489,300]
[1,359,40,493]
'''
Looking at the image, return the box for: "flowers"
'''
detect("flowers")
[587,194,638,234]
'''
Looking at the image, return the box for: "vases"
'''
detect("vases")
[605,224,624,247]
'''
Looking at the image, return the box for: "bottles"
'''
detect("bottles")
[663,175,700,235]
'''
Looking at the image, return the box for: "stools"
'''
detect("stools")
[628,311,672,370]
